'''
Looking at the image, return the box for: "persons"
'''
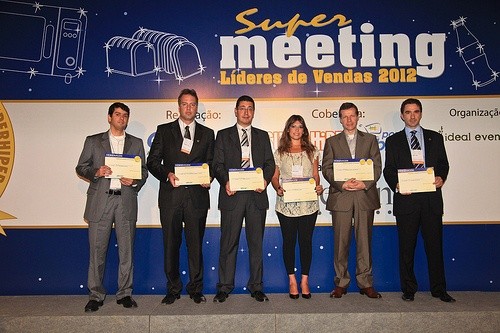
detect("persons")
[212,96,276,303]
[271,115,323,300]
[321,103,382,299]
[147,88,215,305]
[76,102,148,313]
[383,98,456,303]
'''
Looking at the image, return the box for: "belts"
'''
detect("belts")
[108,189,122,195]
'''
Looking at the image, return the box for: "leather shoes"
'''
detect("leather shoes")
[84,300,104,312]
[251,292,268,302]
[435,293,456,303]
[117,295,137,308]
[330,288,346,298]
[360,286,382,298]
[402,290,415,301]
[160,294,180,305]
[213,291,228,303]
[190,293,207,305]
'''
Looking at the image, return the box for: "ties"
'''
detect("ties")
[241,128,250,168]
[410,131,424,169]
[184,126,191,139]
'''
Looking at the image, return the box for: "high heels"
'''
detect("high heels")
[300,280,311,300]
[289,293,300,299]
